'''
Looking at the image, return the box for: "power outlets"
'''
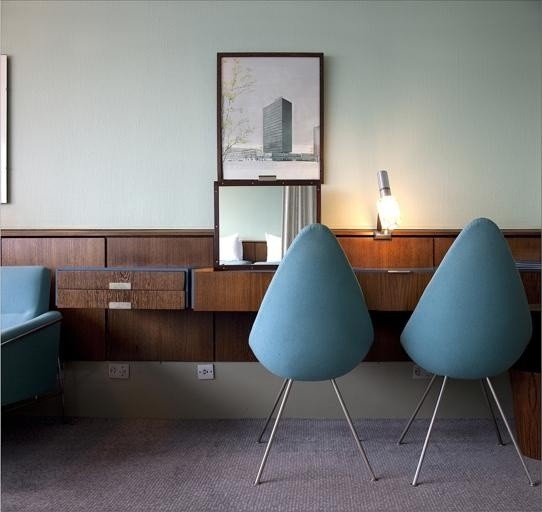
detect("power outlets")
[108,363,130,378]
[197,364,215,379]
[412,365,432,380]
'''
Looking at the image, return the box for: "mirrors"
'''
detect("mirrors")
[213,181,321,270]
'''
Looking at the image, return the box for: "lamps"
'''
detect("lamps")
[373,170,398,240]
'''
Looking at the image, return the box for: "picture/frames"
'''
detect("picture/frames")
[216,51,324,185]
[0,54,8,203]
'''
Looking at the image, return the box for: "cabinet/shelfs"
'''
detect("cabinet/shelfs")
[241,241,267,263]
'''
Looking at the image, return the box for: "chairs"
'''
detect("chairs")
[396,216,536,489]
[0,265,63,408]
[247,222,378,486]
